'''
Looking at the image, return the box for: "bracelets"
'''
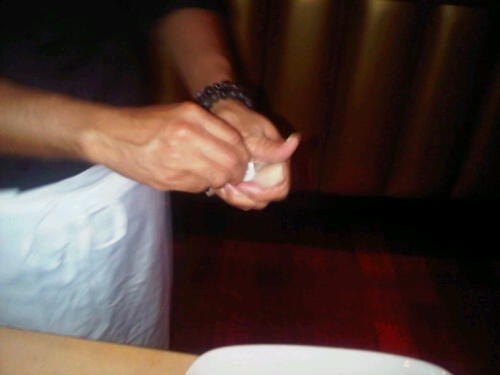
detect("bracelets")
[195,78,252,112]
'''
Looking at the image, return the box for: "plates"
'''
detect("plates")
[184,344,450,375]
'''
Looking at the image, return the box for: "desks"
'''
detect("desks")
[0,326,198,375]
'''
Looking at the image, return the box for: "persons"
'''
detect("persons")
[0,0,300,352]
